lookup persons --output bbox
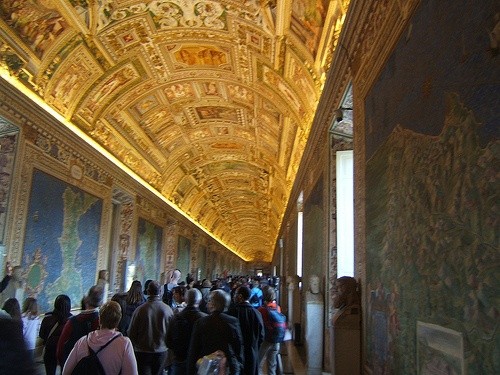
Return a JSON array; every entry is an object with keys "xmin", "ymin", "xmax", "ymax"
[
  {"xmin": 97, "ymin": 270, "xmax": 109, "ymax": 304},
  {"xmin": 9, "ymin": 266, "xmax": 25, "ymax": 312},
  {"xmin": 0, "ymin": 262, "xmax": 286, "ymax": 375},
  {"xmin": 330, "ymin": 276, "xmax": 362, "ymax": 375},
  {"xmin": 286, "ymin": 275, "xmax": 301, "ymax": 328}
]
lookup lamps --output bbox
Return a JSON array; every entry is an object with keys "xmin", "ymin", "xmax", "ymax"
[{"xmin": 336, "ymin": 105, "xmax": 353, "ymax": 121}]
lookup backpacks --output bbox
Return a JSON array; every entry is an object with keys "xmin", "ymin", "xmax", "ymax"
[
  {"xmin": 250, "ymin": 289, "xmax": 260, "ymax": 303},
  {"xmin": 63, "ymin": 315, "xmax": 97, "ymax": 361},
  {"xmin": 70, "ymin": 333, "xmax": 122, "ymax": 374},
  {"xmin": 263, "ymin": 303, "xmax": 285, "ymax": 344},
  {"xmin": 162, "ymin": 283, "xmax": 175, "ymax": 307}
]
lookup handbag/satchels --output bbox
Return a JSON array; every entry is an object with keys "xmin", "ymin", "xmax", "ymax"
[{"xmin": 40, "ymin": 345, "xmax": 46, "ymax": 363}]
